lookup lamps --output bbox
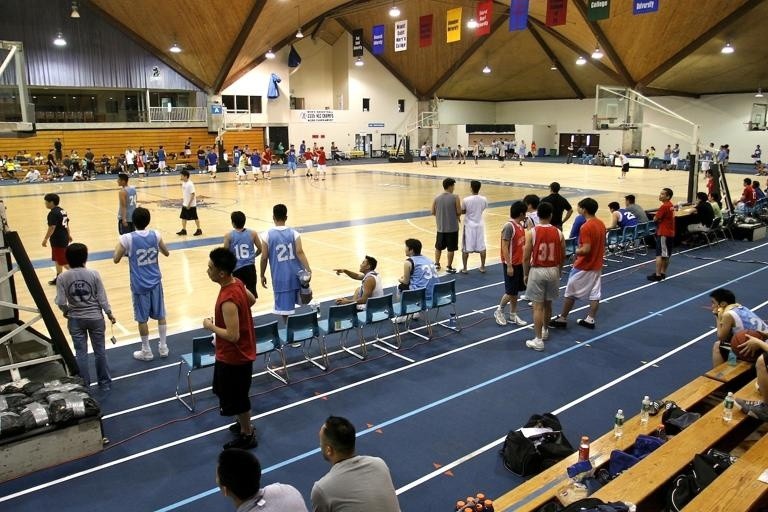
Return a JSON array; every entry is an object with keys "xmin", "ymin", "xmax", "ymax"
[{"xmin": 556, "ymin": 194, "xmax": 767, "ymax": 274}]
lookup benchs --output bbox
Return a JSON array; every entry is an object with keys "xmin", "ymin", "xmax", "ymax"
[
  {"xmin": 0, "ymin": 125, "xmax": 267, "ymax": 180},
  {"xmin": 644, "ymin": 158, "xmax": 764, "ymax": 176}
]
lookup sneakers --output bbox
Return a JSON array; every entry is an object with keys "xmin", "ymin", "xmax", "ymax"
[
  {"xmin": 133, "ymin": 349, "xmax": 153, "ymax": 361},
  {"xmin": 460, "ymin": 267, "xmax": 487, "ymax": 274},
  {"xmin": 494, "ymin": 294, "xmax": 595, "ymax": 352},
  {"xmin": 159, "ymin": 344, "xmax": 169, "ymax": 357},
  {"xmin": 176, "ymin": 229, "xmax": 187, "ymax": 236},
  {"xmin": 159, "ymin": 172, "xmax": 169, "ymax": 175},
  {"xmin": 647, "ymin": 273, "xmax": 665, "ymax": 282},
  {"xmin": 434, "ymin": 263, "xmax": 456, "ymax": 274},
  {"xmin": 48, "ymin": 273, "xmax": 59, "ymax": 285},
  {"xmin": 222, "ymin": 419, "xmax": 258, "ymax": 451},
  {"xmin": 199, "ymin": 169, "xmax": 327, "ymax": 185},
  {"xmin": 193, "ymin": 229, "xmax": 202, "ymax": 236},
  {"xmin": 390, "ymin": 312, "xmax": 420, "ymax": 324}
]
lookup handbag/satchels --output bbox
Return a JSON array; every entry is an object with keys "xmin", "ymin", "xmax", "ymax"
[{"xmin": 502, "ymin": 413, "xmax": 576, "ymax": 477}]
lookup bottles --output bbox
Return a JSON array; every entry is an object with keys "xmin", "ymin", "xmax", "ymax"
[
  {"xmin": 722, "ymin": 392, "xmax": 734, "ymax": 421},
  {"xmin": 578, "ymin": 436, "xmax": 590, "ymax": 462},
  {"xmin": 640, "ymin": 395, "xmax": 650, "ymax": 425},
  {"xmin": 728, "ymin": 349, "xmax": 736, "ymax": 365},
  {"xmin": 613, "ymin": 408, "xmax": 625, "ymax": 438}
]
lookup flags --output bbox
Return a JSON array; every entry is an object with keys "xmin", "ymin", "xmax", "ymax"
[{"xmin": 351, "ymin": 0, "xmax": 659, "ymax": 58}]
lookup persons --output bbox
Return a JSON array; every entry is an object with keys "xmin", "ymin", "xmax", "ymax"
[
  {"xmin": 569, "ymin": 201, "xmax": 586, "ymax": 246},
  {"xmin": 175, "ymin": 170, "xmax": 203, "ymax": 236},
  {"xmin": 41, "ymin": 192, "xmax": 74, "ymax": 286},
  {"xmin": 112, "ymin": 206, "xmax": 170, "ymax": 361},
  {"xmin": 734, "ymin": 329, "xmax": 768, "ymax": 423},
  {"xmin": 215, "ymin": 447, "xmax": 309, "ymax": 512},
  {"xmin": 751, "ymin": 145, "xmax": 768, "ymax": 176},
  {"xmin": 550, "ymin": 197, "xmax": 607, "ymax": 329},
  {"xmin": 734, "ymin": 178, "xmax": 768, "ymax": 214},
  {"xmin": 680, "ymin": 170, "xmax": 726, "ymax": 246},
  {"xmin": 461, "ymin": 180, "xmax": 488, "ymax": 274},
  {"xmin": 260, "ymin": 203, "xmax": 313, "ymax": 348},
  {"xmin": 708, "ymin": 288, "xmax": 768, "ymax": 370},
  {"xmin": 390, "ymin": 238, "xmax": 440, "ymax": 325},
  {"xmin": 309, "ymin": 414, "xmax": 402, "ymax": 511},
  {"xmin": 0, "ymin": 134, "xmax": 343, "ymax": 184},
  {"xmin": 222, "ymin": 210, "xmax": 264, "ymax": 299},
  {"xmin": 116, "ymin": 173, "xmax": 138, "ymax": 258},
  {"xmin": 54, "ymin": 242, "xmax": 117, "ymax": 391},
  {"xmin": 522, "ymin": 202, "xmax": 567, "ymax": 352},
  {"xmin": 431, "ymin": 177, "xmax": 462, "ymax": 274},
  {"xmin": 563, "ymin": 136, "xmax": 731, "ymax": 179},
  {"xmin": 645, "ymin": 188, "xmax": 676, "ymax": 282},
  {"xmin": 519, "ymin": 194, "xmax": 541, "ymax": 307},
  {"xmin": 418, "ymin": 135, "xmax": 536, "ymax": 169},
  {"xmin": 332, "ymin": 255, "xmax": 385, "ymax": 314},
  {"xmin": 493, "ymin": 200, "xmax": 530, "ymax": 327},
  {"xmin": 541, "ymin": 182, "xmax": 574, "ymax": 233},
  {"xmin": 624, "ymin": 194, "xmax": 650, "ymax": 232},
  {"xmin": 202, "ymin": 246, "xmax": 259, "ymax": 449},
  {"xmin": 604, "ymin": 201, "xmax": 638, "ymax": 237}
]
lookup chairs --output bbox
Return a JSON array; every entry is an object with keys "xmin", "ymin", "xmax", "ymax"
[
  {"xmin": 35, "ymin": 110, "xmax": 106, "ymax": 123},
  {"xmin": 570, "ymin": 149, "xmax": 618, "ymax": 167},
  {"xmin": 172, "ymin": 281, "xmax": 463, "ymax": 414}
]
